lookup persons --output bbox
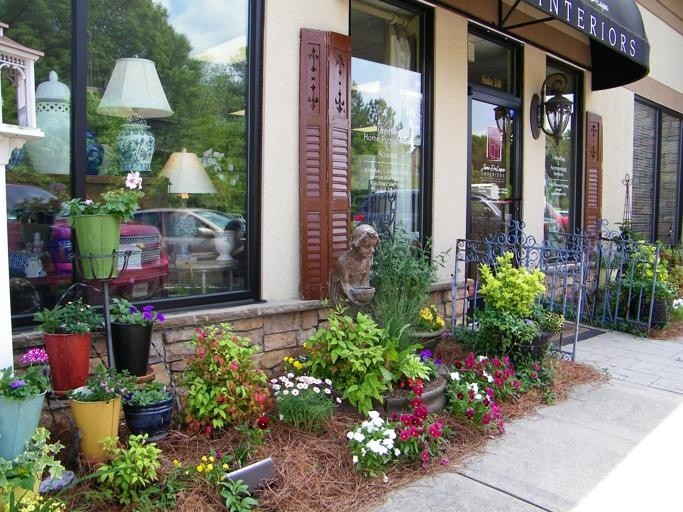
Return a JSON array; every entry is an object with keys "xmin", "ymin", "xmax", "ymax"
[
  {"xmin": 221, "ymin": 220, "xmax": 247, "ymax": 292},
  {"xmin": 329, "ymin": 223, "xmax": 381, "ymax": 320}
]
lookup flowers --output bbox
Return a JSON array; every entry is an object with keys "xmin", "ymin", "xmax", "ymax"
[
  {"xmin": 1, "ymin": 296, "xmax": 170, "ymax": 405},
  {"xmin": 10, "ymin": 182, "xmax": 68, "ymax": 243},
  {"xmin": 393, "ymin": 304, "xmax": 445, "ymax": 393},
  {"xmin": 61, "ymin": 171, "xmax": 146, "ymax": 222}
]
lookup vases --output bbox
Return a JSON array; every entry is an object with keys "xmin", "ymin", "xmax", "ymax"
[
  {"xmin": 2, "ymin": 388, "xmax": 47, "ymax": 460},
  {"xmin": 124, "ymin": 388, "xmax": 176, "ymax": 437},
  {"xmin": 69, "ymin": 398, "xmax": 121, "ymax": 462},
  {"xmin": 72, "ymin": 213, "xmax": 122, "ymax": 279},
  {"xmin": 381, "ymin": 358, "xmax": 449, "ymax": 419},
  {"xmin": 23, "ymin": 231, "xmax": 46, "ymax": 280}
]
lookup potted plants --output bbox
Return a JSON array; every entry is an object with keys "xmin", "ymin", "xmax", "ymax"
[
  {"xmin": 623, "ymin": 239, "xmax": 677, "ymax": 324},
  {"xmin": 476, "ymin": 251, "xmax": 566, "ymax": 366}
]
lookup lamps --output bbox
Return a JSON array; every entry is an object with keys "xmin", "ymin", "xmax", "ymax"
[
  {"xmin": 97, "ymin": 54, "xmax": 174, "ymax": 173},
  {"xmin": 530, "ymin": 72, "xmax": 574, "ymax": 147},
  {"xmin": 157, "ymin": 146, "xmax": 217, "ymax": 262}
]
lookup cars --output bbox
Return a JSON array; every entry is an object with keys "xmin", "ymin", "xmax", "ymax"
[
  {"xmin": 544, "ymin": 200, "xmax": 571, "ymax": 240},
  {"xmin": 363, "ymin": 188, "xmax": 421, "ymax": 243},
  {"xmin": 6, "ymin": 184, "xmax": 246, "ymax": 302},
  {"xmin": 466, "ymin": 183, "xmax": 509, "ymax": 242}
]
